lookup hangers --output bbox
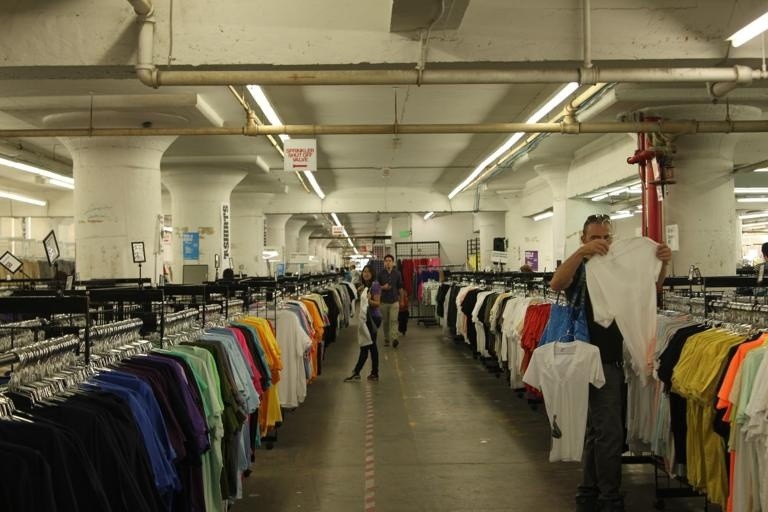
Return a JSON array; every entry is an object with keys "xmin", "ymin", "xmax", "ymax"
[
  {"xmin": 658, "ymin": 287, "xmax": 768, "ymax": 344},
  {"xmin": 598, "ymin": 217, "xmax": 625, "ymax": 250},
  {"xmin": 446, "ymin": 268, "xmax": 568, "ymax": 305},
  {"xmin": 158, "ymin": 309, "xmax": 204, "ymax": 345},
  {"xmin": 223, "ymin": 299, "xmax": 244, "ymax": 319},
  {"xmin": 0, "ymin": 332, "xmax": 100, "ymax": 424},
  {"xmin": 206, "ymin": 304, "xmax": 226, "ymax": 327},
  {"xmin": 551, "ymin": 317, "xmax": 579, "ymax": 358},
  {"xmin": 81, "ymin": 318, "xmax": 153, "ymax": 369}
]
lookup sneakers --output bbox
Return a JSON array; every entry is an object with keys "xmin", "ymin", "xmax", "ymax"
[
  {"xmin": 343, "ymin": 373, "xmax": 362, "ymax": 384},
  {"xmin": 391, "ymin": 338, "xmax": 400, "ymax": 349},
  {"xmin": 366, "ymin": 374, "xmax": 380, "ymax": 382}
]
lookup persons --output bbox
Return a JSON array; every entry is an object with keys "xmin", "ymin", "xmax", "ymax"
[
  {"xmin": 397, "ymin": 289, "xmax": 410, "ymax": 334},
  {"xmin": 520, "ymin": 265, "xmax": 533, "ymax": 272},
  {"xmin": 373, "ymin": 255, "xmax": 403, "ymax": 350},
  {"xmin": 752, "ymin": 242, "xmax": 768, "ymax": 274},
  {"xmin": 328, "ymin": 263, "xmax": 361, "ymax": 286},
  {"xmin": 343, "ymin": 263, "xmax": 381, "ymax": 382},
  {"xmin": 550, "ymin": 214, "xmax": 671, "ymax": 511}
]
